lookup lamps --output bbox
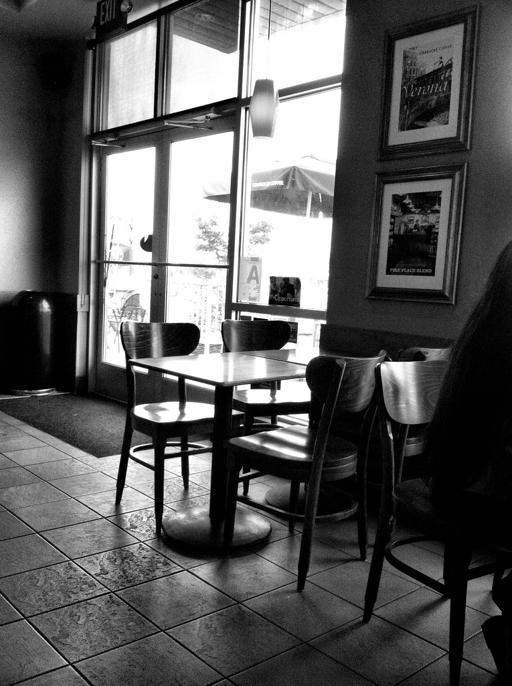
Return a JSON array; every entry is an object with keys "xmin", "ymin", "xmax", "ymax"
[{"xmin": 248, "ymin": 0, "xmax": 281, "ymax": 140}]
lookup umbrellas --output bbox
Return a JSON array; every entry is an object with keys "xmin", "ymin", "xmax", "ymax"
[{"xmin": 197, "ymin": 153, "xmax": 335, "ymax": 222}]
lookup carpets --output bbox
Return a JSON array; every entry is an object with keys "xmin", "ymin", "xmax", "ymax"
[{"xmin": 0, "ymin": 391, "xmax": 210, "ymax": 459}]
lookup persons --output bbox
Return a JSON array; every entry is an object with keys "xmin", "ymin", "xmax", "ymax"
[
  {"xmin": 270, "ymin": 276, "xmax": 300, "ymax": 298},
  {"xmin": 413, "ymin": 219, "xmax": 420, "ymax": 230},
  {"xmin": 419, "ymin": 236, "xmax": 512, "ymax": 686}
]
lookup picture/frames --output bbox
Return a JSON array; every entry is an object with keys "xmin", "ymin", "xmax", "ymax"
[{"xmin": 363, "ymin": 4, "xmax": 482, "ymax": 306}]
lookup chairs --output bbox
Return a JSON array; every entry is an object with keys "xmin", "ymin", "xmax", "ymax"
[{"xmin": 106, "ymin": 294, "xmax": 148, "ymax": 352}]
